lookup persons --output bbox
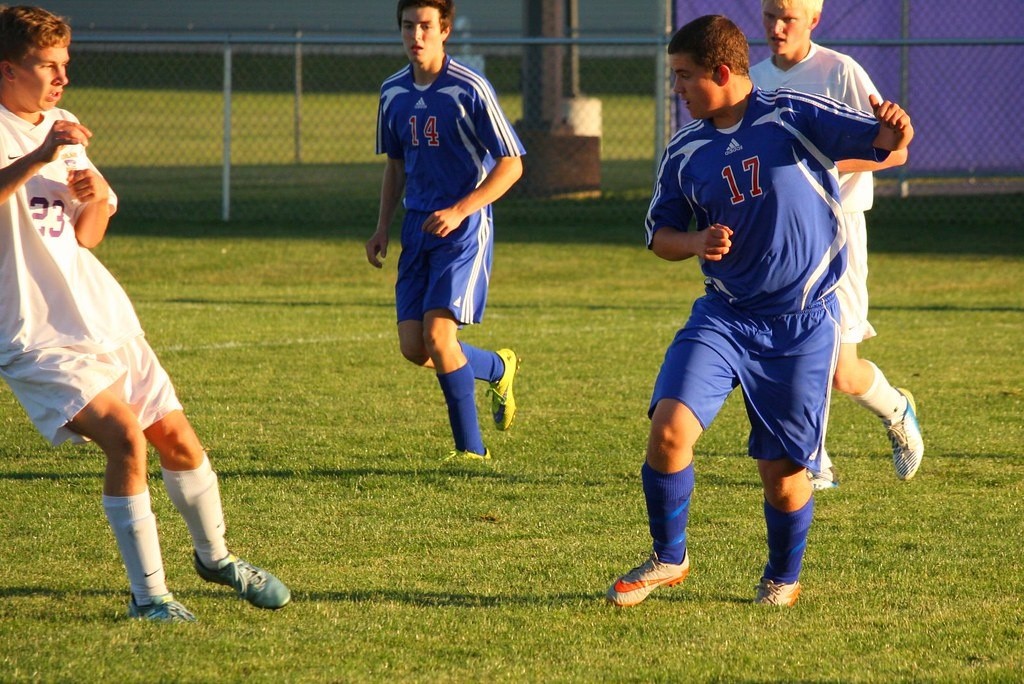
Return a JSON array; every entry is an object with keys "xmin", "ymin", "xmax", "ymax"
[
  {"xmin": 747, "ymin": 0, "xmax": 925, "ymax": 482},
  {"xmin": 364, "ymin": 0, "xmax": 527, "ymax": 469},
  {"xmin": 607, "ymin": 13, "xmax": 915, "ymax": 609},
  {"xmin": 1, "ymin": 5, "xmax": 292, "ymax": 623}
]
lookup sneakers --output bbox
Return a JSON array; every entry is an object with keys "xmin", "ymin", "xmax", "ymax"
[
  {"xmin": 811, "ymin": 448, "xmax": 839, "ymax": 491},
  {"xmin": 883, "ymin": 387, "xmax": 925, "ymax": 480},
  {"xmin": 439, "ymin": 448, "xmax": 492, "ymax": 461},
  {"xmin": 607, "ymin": 549, "xmax": 690, "ymax": 607},
  {"xmin": 193, "ymin": 548, "xmax": 290, "ymax": 609},
  {"xmin": 754, "ymin": 575, "xmax": 801, "ymax": 605},
  {"xmin": 128, "ymin": 593, "xmax": 196, "ymax": 623},
  {"xmin": 485, "ymin": 348, "xmax": 520, "ymax": 432}
]
